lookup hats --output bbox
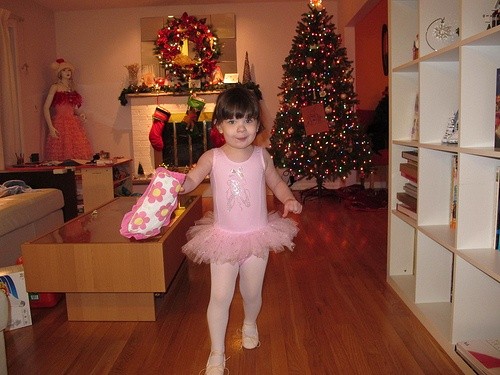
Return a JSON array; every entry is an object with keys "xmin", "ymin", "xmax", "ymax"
[{"xmin": 57, "ymin": 62, "xmax": 74, "ymax": 79}]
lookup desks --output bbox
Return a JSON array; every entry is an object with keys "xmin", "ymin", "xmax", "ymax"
[
  {"xmin": 360, "ymin": 164, "xmax": 388, "ymax": 199},
  {"xmin": 0, "ymin": 163, "xmax": 112, "ymax": 217},
  {"xmin": 22, "ymin": 196, "xmax": 202, "ymax": 321}
]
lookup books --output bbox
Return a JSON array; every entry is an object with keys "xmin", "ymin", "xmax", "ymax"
[
  {"xmin": 450, "ymin": 154, "xmax": 458, "ymax": 229},
  {"xmin": 494, "ymin": 68, "xmax": 500, "ymax": 148},
  {"xmin": 454, "ymin": 337, "xmax": 500, "ymax": 375},
  {"xmin": 493, "ymin": 166, "xmax": 500, "ymax": 251},
  {"xmin": 396, "ymin": 147, "xmax": 418, "ymax": 221}
]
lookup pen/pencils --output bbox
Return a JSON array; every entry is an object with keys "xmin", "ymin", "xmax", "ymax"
[{"xmin": 15, "ymin": 152, "xmax": 25, "ymax": 157}]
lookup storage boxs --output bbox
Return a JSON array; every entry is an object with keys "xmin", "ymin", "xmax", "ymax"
[{"xmin": 0, "ymin": 258, "xmax": 59, "ymax": 331}]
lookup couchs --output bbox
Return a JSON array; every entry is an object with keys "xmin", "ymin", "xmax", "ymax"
[{"xmin": 0, "ymin": 188, "xmax": 64, "ymax": 268}]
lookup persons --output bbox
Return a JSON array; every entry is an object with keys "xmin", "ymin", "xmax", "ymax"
[
  {"xmin": 43, "ymin": 58, "xmax": 95, "ymax": 160},
  {"xmin": 177, "ymin": 81, "xmax": 303, "ymax": 375}
]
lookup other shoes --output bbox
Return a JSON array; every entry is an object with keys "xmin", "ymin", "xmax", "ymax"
[
  {"xmin": 205, "ymin": 351, "xmax": 226, "ymax": 375},
  {"xmin": 242, "ymin": 323, "xmax": 258, "ymax": 349}
]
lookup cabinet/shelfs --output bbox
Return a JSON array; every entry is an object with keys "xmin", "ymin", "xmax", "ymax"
[
  {"xmin": 130, "ymin": 88, "xmax": 221, "ymax": 196},
  {"xmin": 111, "ymin": 156, "xmax": 133, "ymax": 195},
  {"xmin": 387, "ymin": 0, "xmax": 500, "ymax": 375}
]
